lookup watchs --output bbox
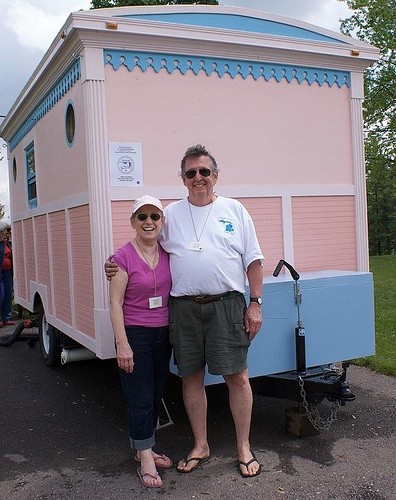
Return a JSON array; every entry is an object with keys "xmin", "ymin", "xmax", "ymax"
[{"xmin": 249, "ymin": 297, "xmax": 262, "ymax": 305}]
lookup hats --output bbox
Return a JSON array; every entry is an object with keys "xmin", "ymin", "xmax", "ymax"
[{"xmin": 131, "ymin": 195, "xmax": 162, "ymax": 213}]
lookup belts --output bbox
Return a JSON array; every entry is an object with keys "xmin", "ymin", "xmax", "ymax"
[{"xmin": 179, "ymin": 291, "xmax": 238, "ymax": 303}]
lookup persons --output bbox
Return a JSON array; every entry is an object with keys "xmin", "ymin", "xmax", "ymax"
[
  {"xmin": 105, "ymin": 145, "xmax": 265, "ymax": 478},
  {"xmin": 109, "ymin": 195, "xmax": 172, "ymax": 487},
  {"xmin": 0, "ymin": 218, "xmax": 16, "ymax": 327}
]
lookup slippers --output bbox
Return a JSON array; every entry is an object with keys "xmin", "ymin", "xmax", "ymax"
[
  {"xmin": 136, "ymin": 466, "xmax": 163, "ymax": 488},
  {"xmin": 237, "ymin": 449, "xmax": 260, "ymax": 477},
  {"xmin": 175, "ymin": 449, "xmax": 212, "ymax": 473},
  {"xmin": 133, "ymin": 452, "xmax": 172, "ymax": 467}
]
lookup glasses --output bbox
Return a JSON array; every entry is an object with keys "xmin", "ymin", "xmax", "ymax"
[
  {"xmin": 137, "ymin": 214, "xmax": 160, "ymax": 220},
  {"xmin": 184, "ymin": 168, "xmax": 210, "ymax": 179}
]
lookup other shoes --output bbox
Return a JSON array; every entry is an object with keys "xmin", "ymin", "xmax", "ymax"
[{"xmin": 0, "ymin": 320, "xmax": 15, "ymax": 326}]
